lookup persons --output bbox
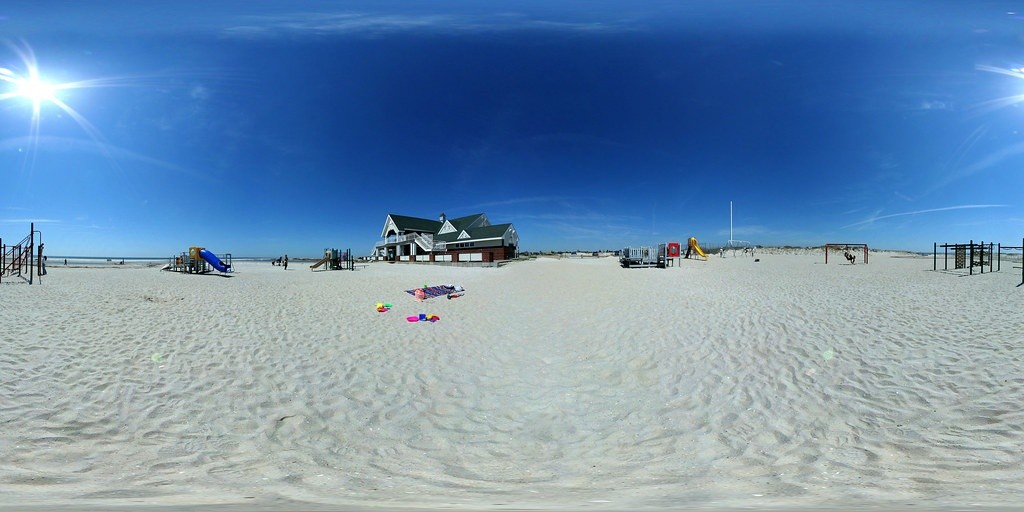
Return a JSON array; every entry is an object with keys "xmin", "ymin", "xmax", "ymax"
[
  {"xmin": 845, "ymin": 248, "xmax": 856, "ymax": 263},
  {"xmin": 42, "ymin": 255, "xmax": 47, "ymax": 275},
  {"xmin": 64, "ymin": 259, "xmax": 68, "ymax": 265},
  {"xmin": 277, "ymin": 257, "xmax": 282, "ymax": 266},
  {"xmin": 284, "ymin": 255, "xmax": 288, "ymax": 270},
  {"xmin": 719, "ymin": 247, "xmax": 757, "ymax": 258}
]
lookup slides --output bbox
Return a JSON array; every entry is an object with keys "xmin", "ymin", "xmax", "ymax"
[
  {"xmin": 694, "ymin": 244, "xmax": 709, "ymax": 257},
  {"xmin": 310, "ymin": 258, "xmax": 329, "ymax": 268},
  {"xmin": 199, "ymin": 249, "xmax": 231, "ymax": 272}
]
